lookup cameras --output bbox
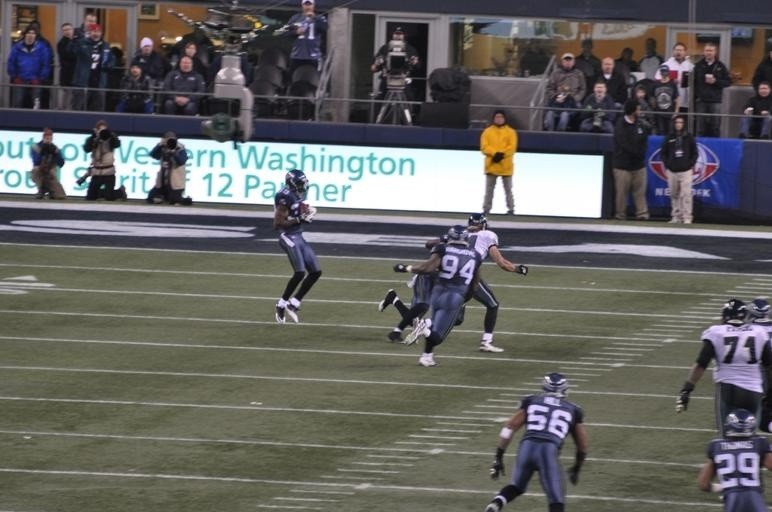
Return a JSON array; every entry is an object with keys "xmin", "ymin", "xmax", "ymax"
[
  {"xmin": 40, "ymin": 143, "xmax": 54, "ymax": 154},
  {"xmin": 96, "ymin": 128, "xmax": 112, "ymax": 143},
  {"xmin": 76, "ymin": 172, "xmax": 91, "ymax": 186},
  {"xmin": 167, "ymin": 137, "xmax": 178, "ymax": 150}
]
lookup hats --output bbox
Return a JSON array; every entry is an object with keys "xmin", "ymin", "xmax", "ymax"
[
  {"xmin": 140, "ymin": 36, "xmax": 154, "ymax": 49},
  {"xmin": 658, "ymin": 65, "xmax": 670, "ymax": 73},
  {"xmin": 41, "ymin": 126, "xmax": 53, "ymax": 136},
  {"xmin": 491, "ymin": 109, "xmax": 507, "ymax": 119},
  {"xmin": 95, "ymin": 119, "xmax": 108, "ymax": 128},
  {"xmin": 391, "ymin": 25, "xmax": 404, "ymax": 35},
  {"xmin": 163, "ymin": 131, "xmax": 177, "ymax": 140},
  {"xmin": 559, "ymin": 53, "xmax": 575, "ymax": 60},
  {"xmin": 88, "ymin": 23, "xmax": 102, "ymax": 33},
  {"xmin": 300, "ymin": 0, "xmax": 315, "ymax": 5}
]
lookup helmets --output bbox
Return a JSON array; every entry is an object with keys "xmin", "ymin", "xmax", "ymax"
[
  {"xmin": 541, "ymin": 370, "xmax": 569, "ymax": 392},
  {"xmin": 448, "ymin": 225, "xmax": 466, "ymax": 244},
  {"xmin": 722, "ymin": 299, "xmax": 748, "ymax": 323},
  {"xmin": 465, "ymin": 212, "xmax": 488, "ymax": 225},
  {"xmin": 748, "ymin": 298, "xmax": 771, "ymax": 319},
  {"xmin": 722, "ymin": 407, "xmax": 756, "ymax": 437},
  {"xmin": 285, "ymin": 170, "xmax": 310, "ymax": 199}
]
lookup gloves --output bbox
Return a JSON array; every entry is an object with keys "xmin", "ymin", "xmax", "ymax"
[
  {"xmin": 493, "ymin": 151, "xmax": 504, "ymax": 162},
  {"xmin": 490, "ymin": 460, "xmax": 507, "ymax": 478},
  {"xmin": 517, "ymin": 262, "xmax": 529, "ymax": 277},
  {"xmin": 302, "ymin": 211, "xmax": 316, "ymax": 223},
  {"xmin": 567, "ymin": 465, "xmax": 578, "ymax": 487},
  {"xmin": 392, "ymin": 262, "xmax": 408, "ymax": 273},
  {"xmin": 675, "ymin": 392, "xmax": 689, "ymax": 414},
  {"xmin": 301, "ymin": 202, "xmax": 317, "ymax": 211}
]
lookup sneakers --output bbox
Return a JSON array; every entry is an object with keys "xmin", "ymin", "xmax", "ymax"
[
  {"xmin": 480, "ymin": 211, "xmax": 490, "ymax": 217},
  {"xmin": 286, "ymin": 299, "xmax": 300, "ymax": 325},
  {"xmin": 505, "ymin": 210, "xmax": 515, "ymax": 215},
  {"xmin": 484, "ymin": 496, "xmax": 502, "ymax": 511},
  {"xmin": 120, "ymin": 185, "xmax": 128, "ymax": 201},
  {"xmin": 388, "ymin": 330, "xmax": 405, "ymax": 346},
  {"xmin": 184, "ymin": 196, "xmax": 193, "ymax": 206},
  {"xmin": 377, "ymin": 286, "xmax": 397, "ymax": 313},
  {"xmin": 403, "ymin": 318, "xmax": 429, "ymax": 346},
  {"xmin": 667, "ymin": 217, "xmax": 681, "ymax": 224},
  {"xmin": 274, "ymin": 303, "xmax": 286, "ymax": 324},
  {"xmin": 682, "ymin": 218, "xmax": 691, "ymax": 225},
  {"xmin": 611, "ymin": 215, "xmax": 627, "ymax": 220},
  {"xmin": 480, "ymin": 338, "xmax": 504, "ymax": 354},
  {"xmin": 633, "ymin": 214, "xmax": 650, "ymax": 221},
  {"xmin": 419, "ymin": 355, "xmax": 442, "ymax": 366},
  {"xmin": 48, "ymin": 191, "xmax": 52, "ymax": 200},
  {"xmin": 35, "ymin": 193, "xmax": 46, "ymax": 199}
]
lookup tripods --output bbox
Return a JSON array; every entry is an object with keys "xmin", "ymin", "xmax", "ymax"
[{"xmin": 376, "ymin": 90, "xmax": 413, "ymax": 125}]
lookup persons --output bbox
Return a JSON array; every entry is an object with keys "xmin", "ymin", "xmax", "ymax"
[
  {"xmin": 379, "ymin": 235, "xmax": 456, "ymax": 344},
  {"xmin": 29, "ymin": 127, "xmax": 68, "ymax": 199},
  {"xmin": 72, "ymin": 25, "xmax": 115, "ymax": 111},
  {"xmin": 7, "ymin": 26, "xmax": 50, "ymax": 108},
  {"xmin": 676, "ymin": 300, "xmax": 771, "ymax": 439},
  {"xmin": 589, "ymin": 57, "xmax": 628, "ymax": 107},
  {"xmin": 633, "ymin": 83, "xmax": 655, "ymax": 133},
  {"xmin": 660, "ymin": 116, "xmax": 698, "ymax": 224},
  {"xmin": 274, "ymin": 170, "xmax": 322, "ymax": 326},
  {"xmin": 163, "ymin": 56, "xmax": 206, "ymax": 115},
  {"xmin": 370, "ymin": 25, "xmax": 419, "ymax": 115},
  {"xmin": 746, "ymin": 294, "xmax": 771, "ymax": 431},
  {"xmin": 638, "ymin": 38, "xmax": 665, "ymax": 75},
  {"xmin": 9, "ymin": 20, "xmax": 54, "ymax": 108},
  {"xmin": 57, "ymin": 22, "xmax": 76, "ymax": 87},
  {"xmin": 425, "ymin": 214, "xmax": 528, "ymax": 353},
  {"xmin": 132, "ymin": 37, "xmax": 166, "ymax": 82},
  {"xmin": 65, "ymin": 13, "xmax": 99, "ymax": 69},
  {"xmin": 147, "ymin": 132, "xmax": 194, "ymax": 205},
  {"xmin": 695, "ymin": 41, "xmax": 732, "ymax": 136},
  {"xmin": 393, "ymin": 225, "xmax": 481, "ymax": 367},
  {"xmin": 614, "ymin": 48, "xmax": 640, "ymax": 70},
  {"xmin": 168, "ymin": 41, "xmax": 206, "ymax": 79},
  {"xmin": 486, "ymin": 374, "xmax": 588, "ymax": 512},
  {"xmin": 612, "ymin": 100, "xmax": 652, "ymax": 221},
  {"xmin": 542, "ymin": 52, "xmax": 587, "ymax": 131},
  {"xmin": 739, "ymin": 82, "xmax": 770, "ymax": 138},
  {"xmin": 481, "ymin": 110, "xmax": 519, "ymax": 215},
  {"xmin": 699, "ymin": 408, "xmax": 772, "ymax": 512},
  {"xmin": 751, "ymin": 49, "xmax": 771, "ymax": 89},
  {"xmin": 652, "ymin": 65, "xmax": 680, "ymax": 135},
  {"xmin": 84, "ymin": 119, "xmax": 127, "ymax": 199},
  {"xmin": 580, "ymin": 80, "xmax": 617, "ymax": 132},
  {"xmin": 573, "ymin": 39, "xmax": 602, "ymax": 79},
  {"xmin": 212, "ymin": 52, "xmax": 253, "ymax": 87},
  {"xmin": 661, "ymin": 41, "xmax": 696, "ymax": 131},
  {"xmin": 115, "ymin": 57, "xmax": 155, "ymax": 113},
  {"xmin": 272, "ymin": 0, "xmax": 328, "ymax": 109}
]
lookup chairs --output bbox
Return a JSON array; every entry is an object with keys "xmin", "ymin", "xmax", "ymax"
[{"xmin": 198, "ymin": 33, "xmax": 323, "ymax": 122}]
